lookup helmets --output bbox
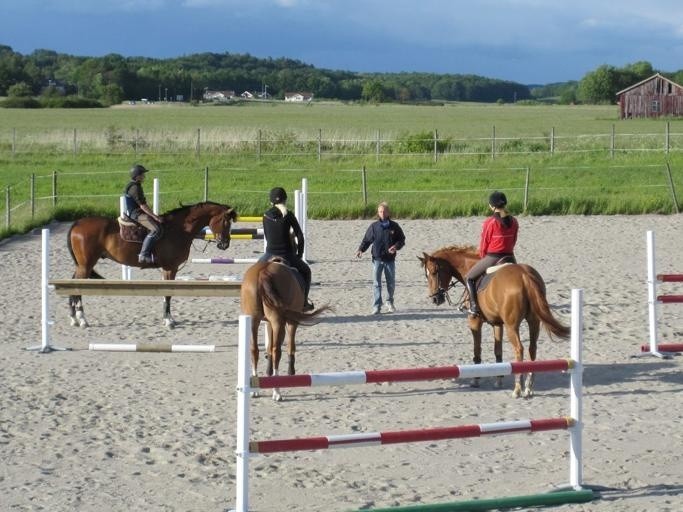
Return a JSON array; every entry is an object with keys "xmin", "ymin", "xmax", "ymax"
[
  {"xmin": 129, "ymin": 164, "xmax": 149, "ymax": 178},
  {"xmin": 269, "ymin": 186, "xmax": 287, "ymax": 204}
]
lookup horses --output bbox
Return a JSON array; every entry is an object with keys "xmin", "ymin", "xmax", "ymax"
[
  {"xmin": 66, "ymin": 199, "xmax": 238, "ymax": 330},
  {"xmin": 415, "ymin": 244, "xmax": 571, "ymax": 400},
  {"xmin": 240, "ymin": 230, "xmax": 337, "ymax": 402}
]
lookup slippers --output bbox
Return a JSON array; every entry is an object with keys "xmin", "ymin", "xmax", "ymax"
[{"xmin": 464, "ymin": 278, "xmax": 479, "ymax": 318}]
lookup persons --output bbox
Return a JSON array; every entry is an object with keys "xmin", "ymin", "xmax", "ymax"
[
  {"xmin": 353, "ymin": 203, "xmax": 405, "ymax": 316},
  {"xmin": 123, "ymin": 163, "xmax": 164, "ymax": 265},
  {"xmin": 465, "ymin": 192, "xmax": 519, "ymax": 317},
  {"xmin": 263, "ymin": 187, "xmax": 314, "ymax": 310}
]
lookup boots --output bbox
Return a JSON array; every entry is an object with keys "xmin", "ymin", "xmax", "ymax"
[
  {"xmin": 304, "ymin": 279, "xmax": 314, "ymax": 311},
  {"xmin": 137, "ymin": 233, "xmax": 153, "ymax": 264}
]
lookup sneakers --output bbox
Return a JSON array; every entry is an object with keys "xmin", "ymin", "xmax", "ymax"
[
  {"xmin": 372, "ymin": 307, "xmax": 381, "ymax": 316},
  {"xmin": 385, "ymin": 301, "xmax": 396, "ymax": 313}
]
